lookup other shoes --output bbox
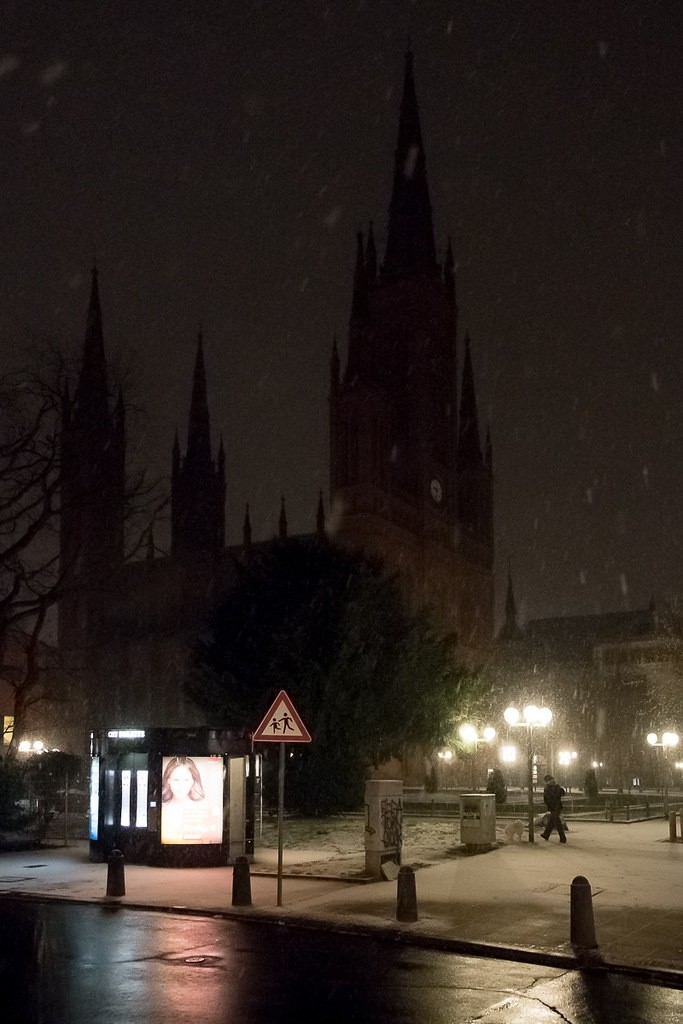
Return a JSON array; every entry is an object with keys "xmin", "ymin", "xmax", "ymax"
[
  {"xmin": 559, "ymin": 838, "xmax": 566, "ymax": 843},
  {"xmin": 540, "ymin": 834, "xmax": 549, "ymax": 840}
]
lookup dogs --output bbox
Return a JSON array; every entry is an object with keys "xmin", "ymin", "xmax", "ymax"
[{"xmin": 504, "ymin": 820, "xmax": 524, "ymax": 843}]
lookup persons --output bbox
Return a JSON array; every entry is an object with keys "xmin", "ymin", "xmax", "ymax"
[{"xmin": 539, "ymin": 775, "xmax": 568, "ymax": 845}]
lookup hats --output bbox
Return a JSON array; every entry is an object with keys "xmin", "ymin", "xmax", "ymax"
[{"xmin": 543, "ymin": 775, "xmax": 553, "ymax": 781}]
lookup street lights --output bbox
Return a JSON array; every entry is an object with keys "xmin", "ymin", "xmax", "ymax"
[
  {"xmin": 648, "ymin": 733, "xmax": 678, "ymax": 818},
  {"xmin": 462, "ymin": 722, "xmax": 496, "ymax": 789},
  {"xmin": 501, "ymin": 705, "xmax": 554, "ymax": 844}
]
